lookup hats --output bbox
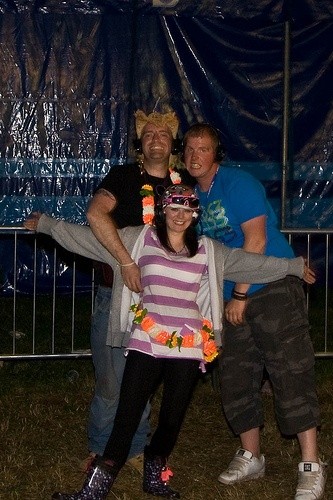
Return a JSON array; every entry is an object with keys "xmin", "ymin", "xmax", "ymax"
[{"xmin": 132, "ymin": 109, "xmax": 180, "ymax": 140}]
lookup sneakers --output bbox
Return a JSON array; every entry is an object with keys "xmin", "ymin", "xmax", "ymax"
[
  {"xmin": 294, "ymin": 456, "xmax": 327, "ymax": 500},
  {"xmin": 217, "ymin": 449, "xmax": 264, "ymax": 485}
]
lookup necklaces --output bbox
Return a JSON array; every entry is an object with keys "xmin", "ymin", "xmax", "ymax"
[
  {"xmin": 136, "ymin": 153, "xmax": 182, "ymax": 224},
  {"xmin": 167, "ymin": 233, "xmax": 186, "ymax": 254},
  {"xmin": 194, "ymin": 163, "xmax": 219, "ymax": 234}
]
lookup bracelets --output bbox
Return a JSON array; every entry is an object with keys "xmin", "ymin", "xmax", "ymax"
[
  {"xmin": 117, "ymin": 260, "xmax": 135, "ymax": 267},
  {"xmin": 231, "ymin": 288, "xmax": 247, "ymax": 301}
]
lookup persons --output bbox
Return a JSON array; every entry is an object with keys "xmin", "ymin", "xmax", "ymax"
[
  {"xmin": 186, "ymin": 123, "xmax": 328, "ymax": 500},
  {"xmin": 80, "ymin": 112, "xmax": 197, "ymax": 482},
  {"xmin": 23, "ymin": 183, "xmax": 315, "ymax": 500}
]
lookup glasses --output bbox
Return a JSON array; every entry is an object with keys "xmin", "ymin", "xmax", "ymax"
[{"xmin": 162, "ymin": 195, "xmax": 200, "ymax": 208}]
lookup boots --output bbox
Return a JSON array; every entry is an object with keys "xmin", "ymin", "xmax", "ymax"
[
  {"xmin": 143, "ymin": 454, "xmax": 180, "ymax": 498},
  {"xmin": 53, "ymin": 463, "xmax": 117, "ymax": 499}
]
